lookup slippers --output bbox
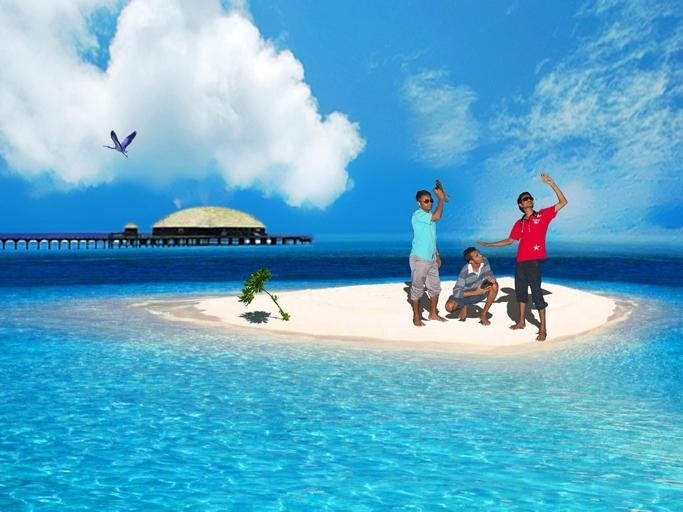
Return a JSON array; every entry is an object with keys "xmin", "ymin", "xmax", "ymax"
[{"xmin": 435, "ymin": 179, "xmax": 449, "ymax": 203}]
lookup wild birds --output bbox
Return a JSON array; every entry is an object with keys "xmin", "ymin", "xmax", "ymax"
[{"xmin": 103, "ymin": 130, "xmax": 136, "ymax": 159}]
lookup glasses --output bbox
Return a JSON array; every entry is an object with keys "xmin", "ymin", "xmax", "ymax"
[
  {"xmin": 419, "ymin": 199, "xmax": 433, "ymax": 204},
  {"xmin": 519, "ymin": 196, "xmax": 534, "ymax": 203}
]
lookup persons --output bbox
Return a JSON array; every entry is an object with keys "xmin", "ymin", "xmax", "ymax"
[
  {"xmin": 445, "ymin": 247, "xmax": 498, "ymax": 326},
  {"xmin": 477, "ymin": 173, "xmax": 568, "ymax": 341},
  {"xmin": 409, "ymin": 189, "xmax": 448, "ymax": 326}
]
